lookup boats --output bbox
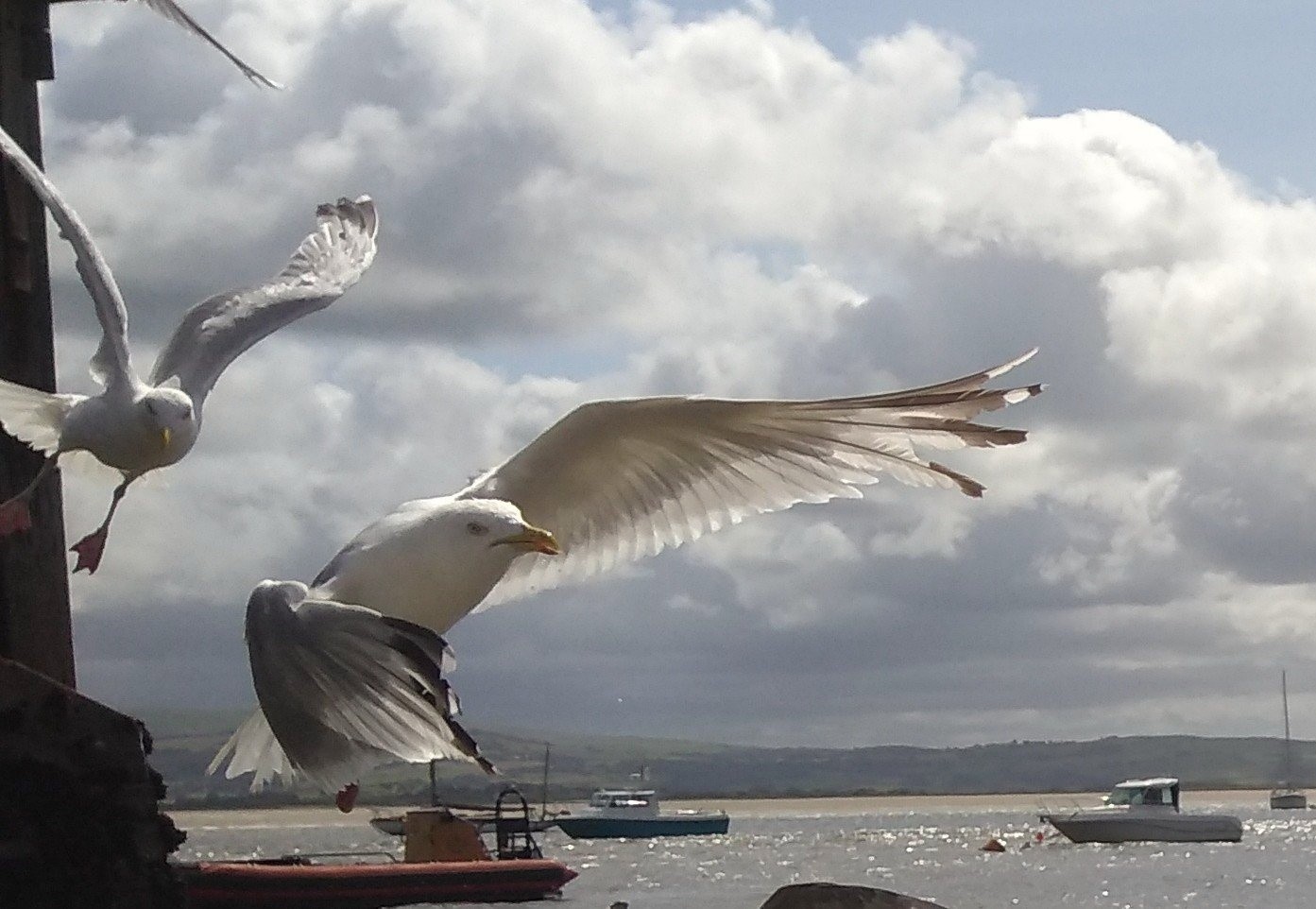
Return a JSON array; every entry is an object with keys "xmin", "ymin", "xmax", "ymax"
[
  {"xmin": 1037, "ymin": 777, "xmax": 1248, "ymax": 845},
  {"xmin": 551, "ymin": 766, "xmax": 731, "ymax": 840},
  {"xmin": 179, "ymin": 784, "xmax": 583, "ymax": 909}
]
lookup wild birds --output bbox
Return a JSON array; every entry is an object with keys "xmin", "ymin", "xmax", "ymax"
[
  {"xmin": 0, "ymin": 118, "xmax": 385, "ymax": 581},
  {"xmin": 198, "ymin": 340, "xmax": 1056, "ymax": 816},
  {"xmin": 1, "ymin": 0, "xmax": 287, "ymax": 94}
]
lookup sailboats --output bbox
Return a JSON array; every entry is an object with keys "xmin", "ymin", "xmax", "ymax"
[
  {"xmin": 368, "ymin": 756, "xmax": 557, "ymax": 841},
  {"xmin": 1268, "ymin": 670, "xmax": 1316, "ymax": 812}
]
